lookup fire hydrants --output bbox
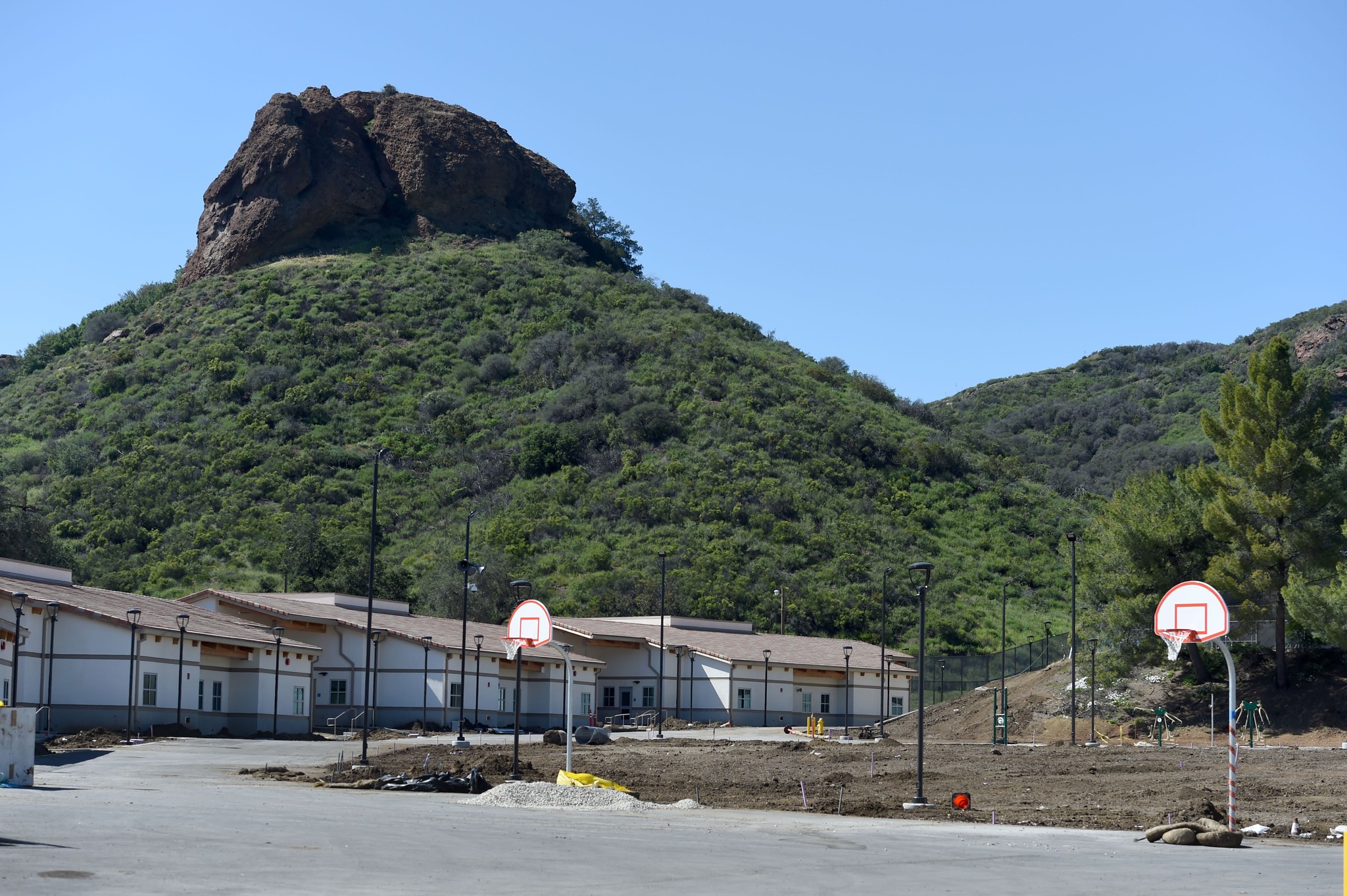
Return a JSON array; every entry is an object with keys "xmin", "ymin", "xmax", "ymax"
[{"xmin": 816, "ymin": 718, "xmax": 825, "ymax": 735}]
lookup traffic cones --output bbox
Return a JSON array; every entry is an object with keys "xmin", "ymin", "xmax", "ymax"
[
  {"xmin": 951, "ymin": 792, "xmax": 971, "ymax": 811},
  {"xmin": 720, "ymin": 720, "xmax": 732, "ymax": 728}
]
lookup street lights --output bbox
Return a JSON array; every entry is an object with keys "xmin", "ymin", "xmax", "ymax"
[
  {"xmin": 1044, "ymin": 621, "xmax": 1052, "ymax": 666},
  {"xmin": 762, "ymin": 649, "xmax": 772, "ymax": 728},
  {"xmin": 906, "ymin": 562, "xmax": 946, "ymax": 804},
  {"xmin": 1000, "ymin": 579, "xmax": 1012, "ymax": 740},
  {"xmin": 1065, "ymin": 532, "xmax": 1100, "ymax": 745},
  {"xmin": 420, "ymin": 635, "xmax": 433, "ymax": 737},
  {"xmin": 877, "ymin": 566, "xmax": 894, "ymax": 739},
  {"xmin": 176, "ymin": 613, "xmax": 191, "ymax": 738},
  {"xmin": 673, "ymin": 646, "xmax": 698, "ymax": 724},
  {"xmin": 273, "ymin": 627, "xmax": 285, "ymax": 740},
  {"xmin": 902, "ymin": 647, "xmax": 910, "ymax": 667},
  {"xmin": 44, "ymin": 602, "xmax": 60, "ymax": 731},
  {"xmin": 126, "ymin": 609, "xmax": 142, "ymax": 746},
  {"xmin": 457, "ymin": 508, "xmax": 489, "ymax": 741},
  {"xmin": 509, "ymin": 580, "xmax": 534, "ymax": 781},
  {"xmin": 558, "ymin": 645, "xmax": 571, "ymax": 731},
  {"xmin": 1026, "ymin": 635, "xmax": 1035, "ymax": 672},
  {"xmin": 656, "ymin": 550, "xmax": 667, "ymax": 738},
  {"xmin": 359, "ymin": 446, "xmax": 393, "ymax": 765},
  {"xmin": 842, "ymin": 645, "xmax": 853, "ymax": 737},
  {"xmin": 773, "ymin": 588, "xmax": 785, "ymax": 635},
  {"xmin": 8, "ymin": 592, "xmax": 29, "ymax": 708}
]
